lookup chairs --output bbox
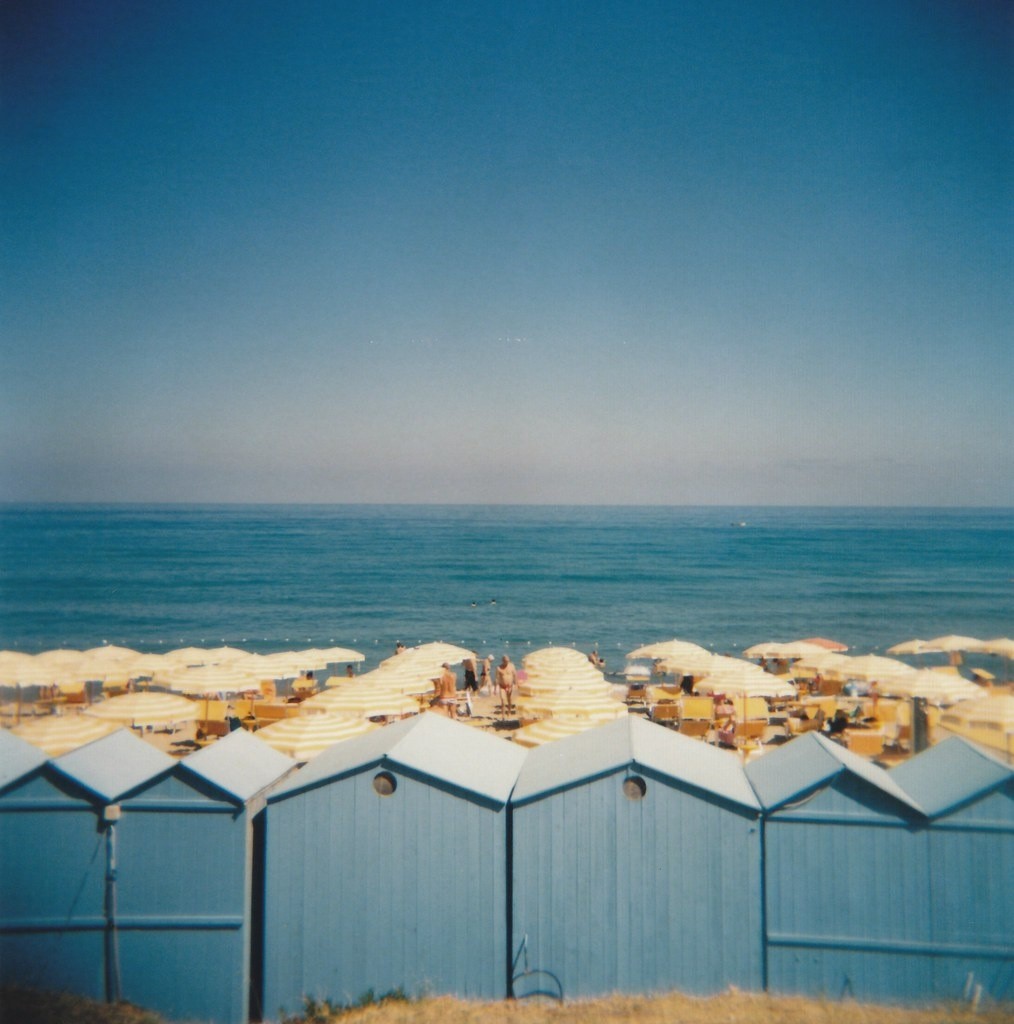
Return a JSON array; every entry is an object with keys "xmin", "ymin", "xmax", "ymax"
[{"xmin": 51, "ymin": 665, "xmax": 934, "ymax": 772}]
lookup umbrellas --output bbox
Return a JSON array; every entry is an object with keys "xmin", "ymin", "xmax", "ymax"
[{"xmin": 0, "ymin": 634, "xmax": 1014, "ymax": 763}]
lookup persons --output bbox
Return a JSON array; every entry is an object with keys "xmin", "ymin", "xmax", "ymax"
[
  {"xmin": 494, "ymin": 656, "xmax": 517, "ymax": 720},
  {"xmin": 440, "ymin": 662, "xmax": 458, "ymax": 719},
  {"xmin": 826, "ymin": 710, "xmax": 847, "ymax": 735},
  {"xmin": 489, "ymin": 600, "xmax": 496, "ymax": 606},
  {"xmin": 773, "ymin": 657, "xmax": 790, "ymax": 672},
  {"xmin": 589, "ymin": 650, "xmax": 597, "ymax": 664},
  {"xmin": 598, "ymin": 658, "xmax": 605, "ymax": 669},
  {"xmin": 462, "ymin": 659, "xmax": 478, "ymax": 717},
  {"xmin": 347, "ymin": 665, "xmax": 353, "ymax": 677},
  {"xmin": 760, "ymin": 656, "xmax": 767, "ymax": 672},
  {"xmin": 396, "ymin": 643, "xmax": 404, "ymax": 654},
  {"xmin": 471, "ymin": 602, "xmax": 478, "ymax": 607},
  {"xmin": 479, "ymin": 654, "xmax": 494, "ymax": 696},
  {"xmin": 225, "ymin": 705, "xmax": 241, "ymax": 732},
  {"xmin": 292, "ymin": 671, "xmax": 316, "ymax": 687}
]
